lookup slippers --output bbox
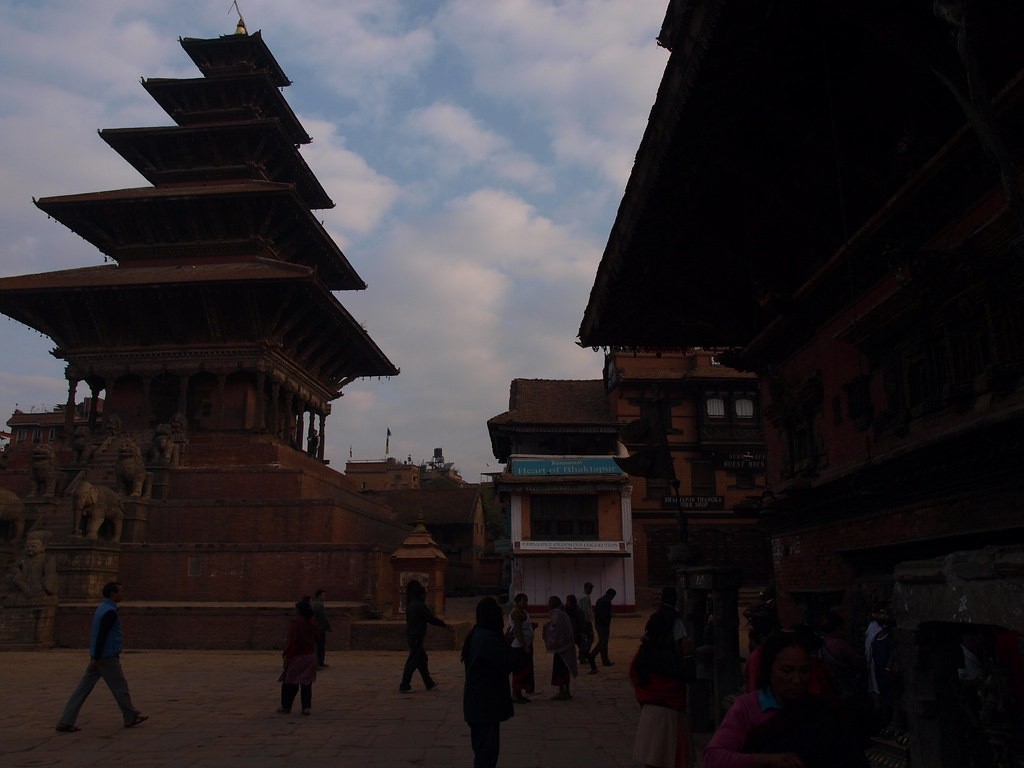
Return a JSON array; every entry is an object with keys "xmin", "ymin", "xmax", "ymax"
[
  {"xmin": 124, "ymin": 715, "xmax": 149, "ymax": 727},
  {"xmin": 55, "ymin": 721, "xmax": 82, "ymax": 733}
]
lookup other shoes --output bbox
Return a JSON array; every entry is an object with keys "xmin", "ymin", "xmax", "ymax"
[
  {"xmin": 276, "ymin": 707, "xmax": 291, "ymax": 714},
  {"xmin": 301, "ymin": 709, "xmax": 310, "ymax": 715},
  {"xmin": 321, "ymin": 664, "xmax": 329, "ymax": 667},
  {"xmin": 550, "ymin": 694, "xmax": 572, "ymax": 701},
  {"xmin": 512, "ymin": 695, "xmax": 532, "ymax": 704},
  {"xmin": 587, "ymin": 668, "xmax": 599, "ymax": 675},
  {"xmin": 399, "ymin": 688, "xmax": 416, "ymax": 693},
  {"xmin": 602, "ymin": 659, "xmax": 615, "ymax": 667}
]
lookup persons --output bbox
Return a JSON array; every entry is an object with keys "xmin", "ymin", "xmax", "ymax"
[
  {"xmin": 590, "ymin": 588, "xmax": 617, "ymax": 666},
  {"xmin": 631, "ymin": 587, "xmax": 874, "ymax": 768},
  {"xmin": 55, "ymin": 581, "xmax": 149, "ymax": 730},
  {"xmin": 543, "ymin": 596, "xmax": 577, "ymax": 700},
  {"xmin": 461, "ymin": 597, "xmax": 523, "ymax": 768},
  {"xmin": 566, "ymin": 583, "xmax": 599, "ymax": 674},
  {"xmin": 275, "ymin": 589, "xmax": 335, "ymax": 716},
  {"xmin": 510, "ymin": 593, "xmax": 538, "ymax": 703},
  {"xmin": 399, "ymin": 579, "xmax": 454, "ymax": 693},
  {"xmin": 701, "ymin": 632, "xmax": 870, "ymax": 768}
]
